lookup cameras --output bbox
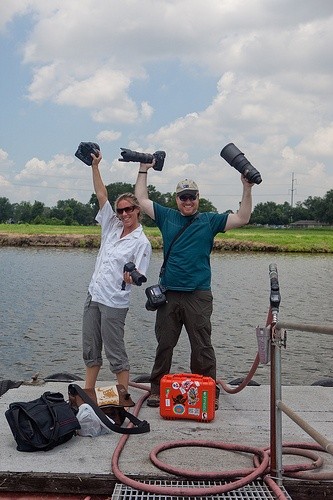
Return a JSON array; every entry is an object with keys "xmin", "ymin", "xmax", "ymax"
[
  {"xmin": 145, "ymin": 285, "xmax": 166, "ymax": 311},
  {"xmin": 74, "ymin": 142, "xmax": 100, "ymax": 166},
  {"xmin": 124, "ymin": 261, "xmax": 147, "ymax": 286},
  {"xmin": 119, "ymin": 147, "xmax": 165, "ymax": 170}
]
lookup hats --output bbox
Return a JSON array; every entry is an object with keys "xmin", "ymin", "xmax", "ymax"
[{"xmin": 176, "ymin": 179, "xmax": 199, "ymax": 193}]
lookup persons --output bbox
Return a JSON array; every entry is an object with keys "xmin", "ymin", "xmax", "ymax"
[
  {"xmin": 134, "ymin": 159, "xmax": 254, "ymax": 411},
  {"xmin": 82, "ymin": 149, "xmax": 152, "ymax": 407}
]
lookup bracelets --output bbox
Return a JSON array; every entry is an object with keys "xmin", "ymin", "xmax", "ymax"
[{"xmin": 139, "ymin": 171, "xmax": 147, "ymax": 175}]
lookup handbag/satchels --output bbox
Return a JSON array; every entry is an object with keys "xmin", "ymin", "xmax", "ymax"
[
  {"xmin": 5, "ymin": 391, "xmax": 81, "ymax": 452},
  {"xmin": 68, "ymin": 383, "xmax": 150, "ymax": 434}
]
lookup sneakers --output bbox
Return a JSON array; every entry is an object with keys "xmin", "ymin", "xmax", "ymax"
[
  {"xmin": 147, "ymin": 390, "xmax": 160, "ymax": 407},
  {"xmin": 215, "ymin": 396, "xmax": 219, "ymax": 410}
]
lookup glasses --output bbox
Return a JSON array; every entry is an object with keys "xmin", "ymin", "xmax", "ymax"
[
  {"xmin": 178, "ymin": 194, "xmax": 197, "ymax": 201},
  {"xmin": 117, "ymin": 205, "xmax": 137, "ymax": 214}
]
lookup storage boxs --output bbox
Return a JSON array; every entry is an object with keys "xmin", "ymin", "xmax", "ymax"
[{"xmin": 159, "ymin": 373, "xmax": 216, "ymax": 422}]
